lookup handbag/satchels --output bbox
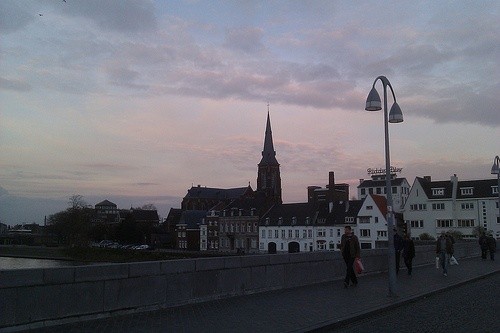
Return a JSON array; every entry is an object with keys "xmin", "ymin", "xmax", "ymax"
[
  {"xmin": 354, "ymin": 260, "xmax": 364, "ymax": 272},
  {"xmin": 436, "ymin": 256, "xmax": 439, "ymax": 268},
  {"xmin": 449, "ymin": 255, "xmax": 458, "ymax": 266}
]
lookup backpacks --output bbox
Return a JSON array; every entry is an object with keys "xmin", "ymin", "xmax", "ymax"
[{"xmin": 482, "ymin": 238, "xmax": 487, "ymax": 246}]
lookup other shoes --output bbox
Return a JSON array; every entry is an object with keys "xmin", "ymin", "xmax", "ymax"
[
  {"xmin": 408, "ymin": 269, "xmax": 412, "ymax": 274},
  {"xmin": 490, "ymin": 258, "xmax": 495, "ymax": 260},
  {"xmin": 443, "ymin": 272, "xmax": 448, "ymax": 276},
  {"xmin": 344, "ymin": 280, "xmax": 349, "ymax": 287},
  {"xmin": 481, "ymin": 254, "xmax": 487, "ymax": 257},
  {"xmin": 351, "ymin": 282, "xmax": 358, "ymax": 287}
]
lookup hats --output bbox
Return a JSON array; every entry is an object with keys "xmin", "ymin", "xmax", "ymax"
[{"xmin": 440, "ymin": 230, "xmax": 448, "ymax": 236}]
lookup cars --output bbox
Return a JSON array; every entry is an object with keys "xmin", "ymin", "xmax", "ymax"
[{"xmin": 90, "ymin": 240, "xmax": 151, "ymax": 250}]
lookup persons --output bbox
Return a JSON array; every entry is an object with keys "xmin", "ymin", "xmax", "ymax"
[
  {"xmin": 340, "ymin": 225, "xmax": 361, "ymax": 288},
  {"xmin": 445, "ymin": 231, "xmax": 454, "ymax": 267},
  {"xmin": 392, "ymin": 228, "xmax": 415, "ymax": 276},
  {"xmin": 435, "ymin": 230, "xmax": 453, "ymax": 277},
  {"xmin": 478, "ymin": 232, "xmax": 490, "ymax": 259},
  {"xmin": 488, "ymin": 234, "xmax": 496, "ymax": 261}
]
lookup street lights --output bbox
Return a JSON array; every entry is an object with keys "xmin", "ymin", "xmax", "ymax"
[
  {"xmin": 491, "ymin": 156, "xmax": 500, "ymax": 219},
  {"xmin": 364, "ymin": 76, "xmax": 405, "ymax": 297}
]
